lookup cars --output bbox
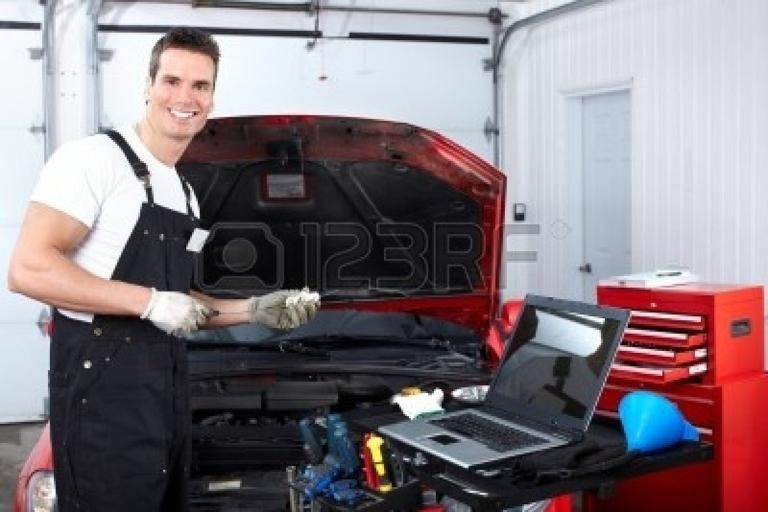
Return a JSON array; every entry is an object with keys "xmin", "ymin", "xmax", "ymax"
[{"xmin": 13, "ymin": 114, "xmax": 526, "ymax": 512}]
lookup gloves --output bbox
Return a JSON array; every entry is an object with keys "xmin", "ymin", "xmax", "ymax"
[
  {"xmin": 139, "ymin": 287, "xmax": 214, "ymax": 337},
  {"xmin": 249, "ymin": 287, "xmax": 322, "ymax": 330}
]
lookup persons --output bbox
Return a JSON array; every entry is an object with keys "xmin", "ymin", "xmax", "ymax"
[{"xmin": 8, "ymin": 26, "xmax": 322, "ymax": 512}]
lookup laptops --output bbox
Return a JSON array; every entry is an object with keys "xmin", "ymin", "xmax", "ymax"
[{"xmin": 378, "ymin": 291, "xmax": 633, "ymax": 470}]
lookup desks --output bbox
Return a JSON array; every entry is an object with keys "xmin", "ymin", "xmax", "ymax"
[{"xmin": 315, "ymin": 404, "xmax": 713, "ymax": 512}]
